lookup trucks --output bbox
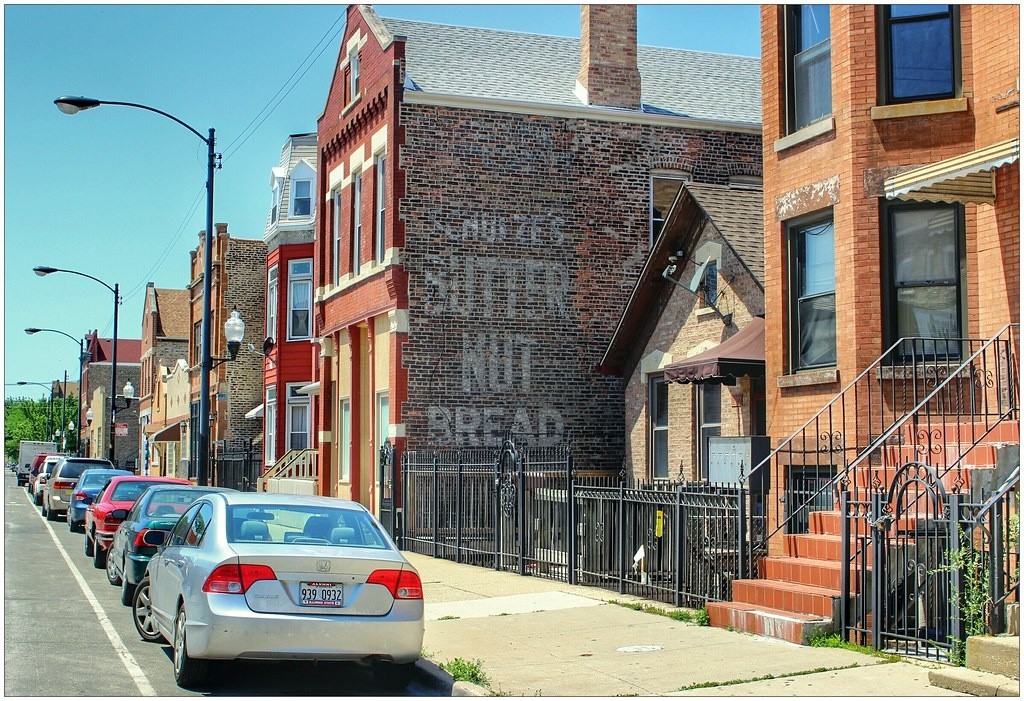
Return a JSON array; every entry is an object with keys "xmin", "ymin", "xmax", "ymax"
[{"xmin": 16, "ymin": 441, "xmax": 57, "ymax": 488}]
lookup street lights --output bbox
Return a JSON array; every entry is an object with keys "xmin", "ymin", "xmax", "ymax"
[
  {"xmin": 53, "ymin": 94, "xmax": 248, "ymax": 485},
  {"xmin": 32, "ymin": 265, "xmax": 126, "ymax": 466},
  {"xmin": 15, "ymin": 379, "xmax": 56, "ymax": 439},
  {"xmin": 22, "ymin": 326, "xmax": 88, "ymax": 458}
]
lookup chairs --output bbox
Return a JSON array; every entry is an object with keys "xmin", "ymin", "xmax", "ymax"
[
  {"xmin": 155, "ymin": 504, "xmax": 176, "ymax": 517},
  {"xmin": 331, "ymin": 526, "xmax": 356, "ymax": 544},
  {"xmin": 235, "ymin": 519, "xmax": 271, "ymax": 541},
  {"xmin": 303, "ymin": 515, "xmax": 340, "ymax": 541}
]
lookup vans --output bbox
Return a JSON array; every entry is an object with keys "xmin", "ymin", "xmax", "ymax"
[
  {"xmin": 41, "ymin": 457, "xmax": 116, "ymax": 520},
  {"xmin": 24, "ymin": 453, "xmax": 67, "ymax": 494}
]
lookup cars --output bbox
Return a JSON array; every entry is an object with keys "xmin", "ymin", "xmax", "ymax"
[
  {"xmin": 30, "ymin": 456, "xmax": 65, "ymax": 506},
  {"xmin": 6, "ymin": 461, "xmax": 19, "ymax": 473},
  {"xmin": 67, "ymin": 469, "xmax": 135, "ymax": 533},
  {"xmin": 132, "ymin": 492, "xmax": 426, "ymax": 689},
  {"xmin": 104, "ymin": 484, "xmax": 245, "ymax": 607},
  {"xmin": 81, "ymin": 477, "xmax": 195, "ymax": 568}
]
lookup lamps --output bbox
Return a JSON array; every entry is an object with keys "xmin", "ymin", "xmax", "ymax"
[
  {"xmin": 209, "ymin": 411, "xmax": 218, "ymax": 427},
  {"xmin": 180, "ymin": 417, "xmax": 191, "ymax": 433}
]
